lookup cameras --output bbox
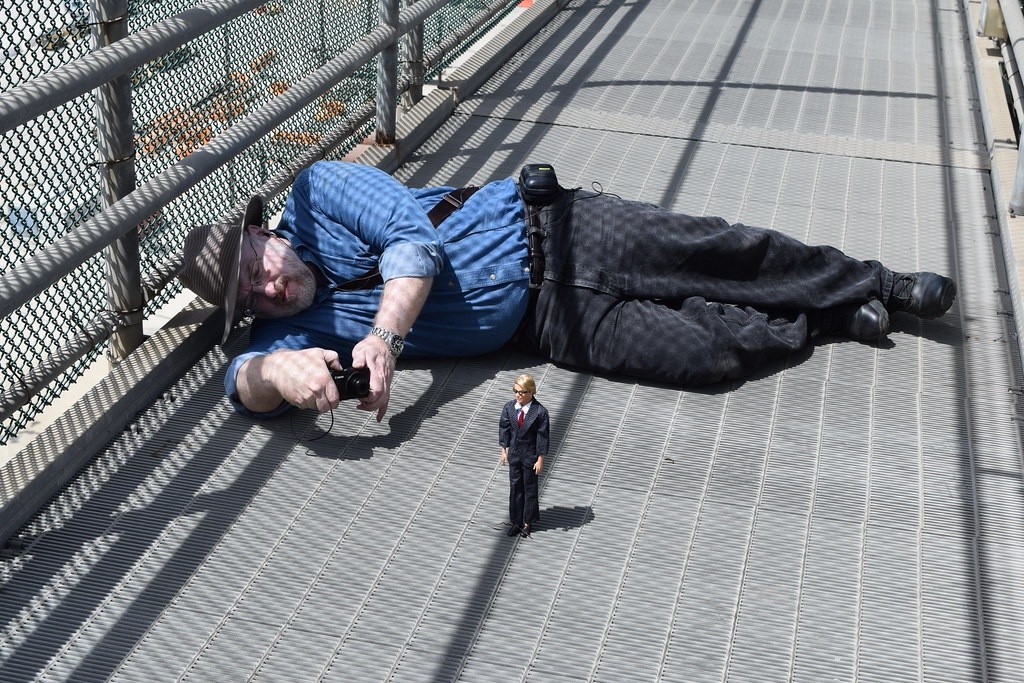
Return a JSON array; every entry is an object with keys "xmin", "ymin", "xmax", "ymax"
[{"xmin": 330, "ymin": 366, "xmax": 370, "ymax": 401}]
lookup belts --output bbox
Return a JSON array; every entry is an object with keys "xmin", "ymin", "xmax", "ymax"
[{"xmin": 506, "ymin": 182, "xmax": 544, "ymax": 348}]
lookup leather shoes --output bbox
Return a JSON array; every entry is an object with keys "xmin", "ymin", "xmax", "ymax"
[
  {"xmin": 833, "ymin": 295, "xmax": 890, "ymax": 341},
  {"xmin": 888, "ymin": 271, "xmax": 957, "ymax": 320}
]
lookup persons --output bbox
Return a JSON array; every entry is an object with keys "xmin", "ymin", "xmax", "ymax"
[
  {"xmin": 178, "ymin": 161, "xmax": 957, "ymax": 424},
  {"xmin": 498, "ymin": 373, "xmax": 550, "ymax": 537}
]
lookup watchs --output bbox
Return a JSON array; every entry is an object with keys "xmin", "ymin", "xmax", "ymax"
[{"xmin": 369, "ymin": 327, "xmax": 405, "ymax": 357}]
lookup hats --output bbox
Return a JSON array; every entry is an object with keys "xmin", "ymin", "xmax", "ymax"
[{"xmin": 178, "ymin": 195, "xmax": 263, "ymax": 351}]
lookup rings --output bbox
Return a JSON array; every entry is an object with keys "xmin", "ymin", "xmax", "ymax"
[{"xmin": 385, "ymin": 393, "xmax": 390, "ymax": 401}]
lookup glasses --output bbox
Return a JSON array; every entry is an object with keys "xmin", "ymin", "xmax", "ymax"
[{"xmin": 239, "ymin": 230, "xmax": 268, "ymax": 318}]
